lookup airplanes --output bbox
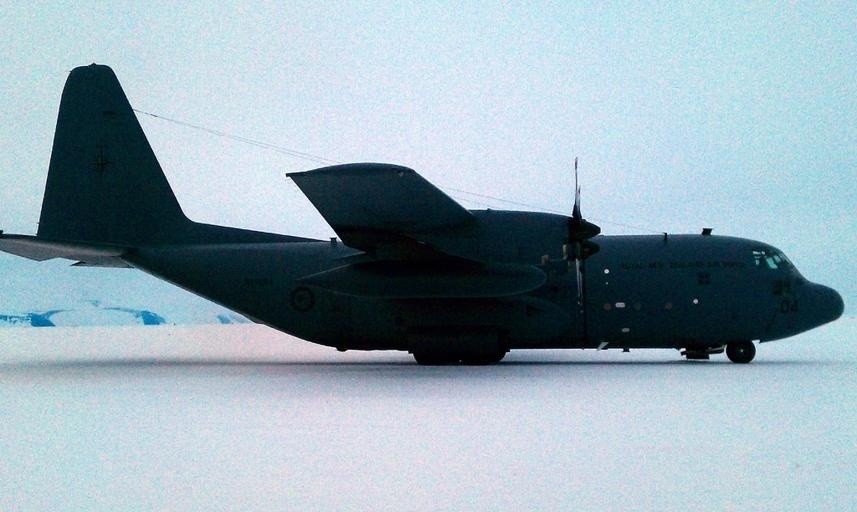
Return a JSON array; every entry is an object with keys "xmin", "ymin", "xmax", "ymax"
[{"xmin": 0, "ymin": 63, "xmax": 844, "ymax": 366}]
